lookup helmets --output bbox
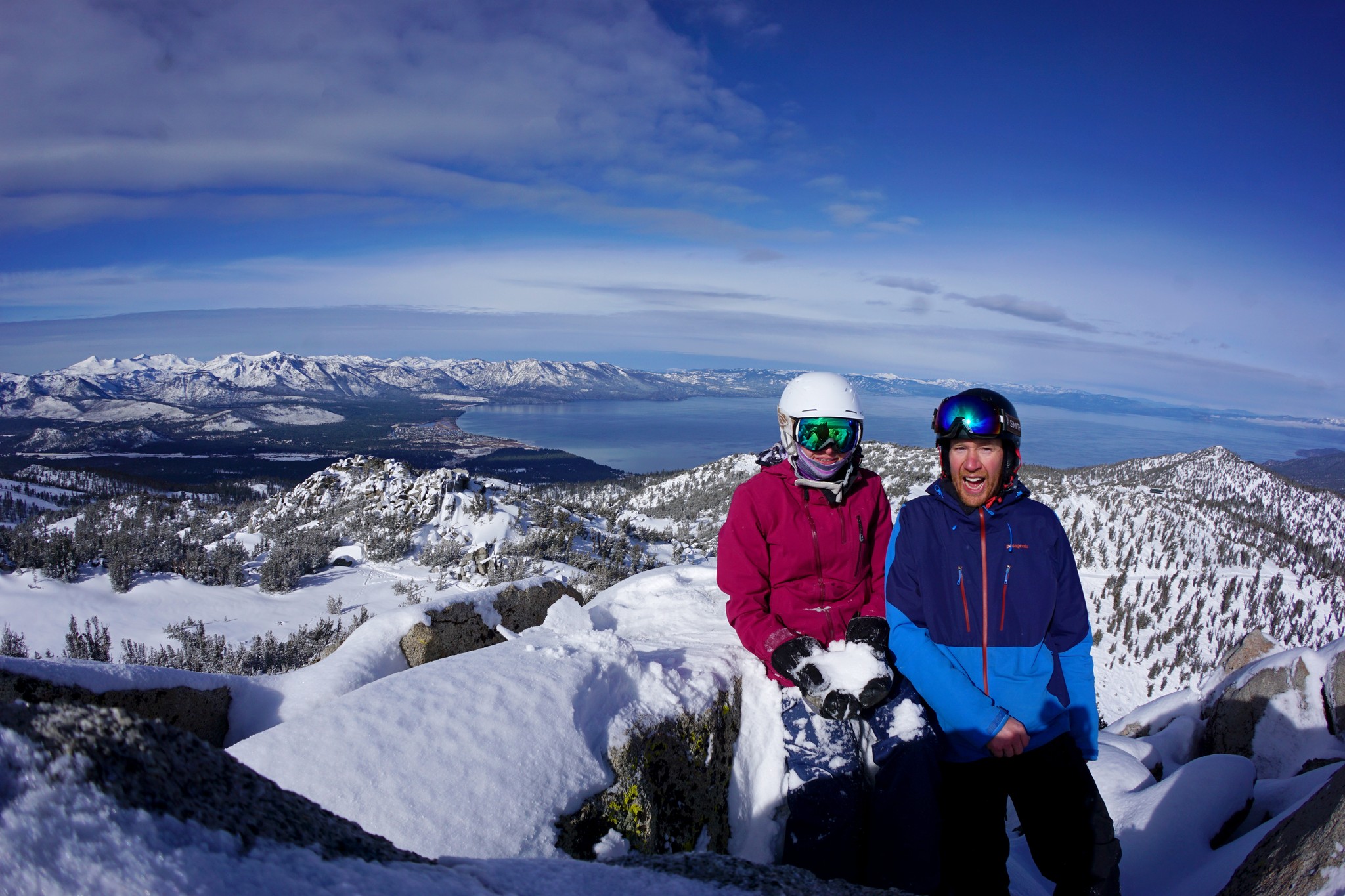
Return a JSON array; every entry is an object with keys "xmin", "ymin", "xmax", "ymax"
[
  {"xmin": 928, "ymin": 387, "xmax": 1021, "ymax": 489},
  {"xmin": 777, "ymin": 372, "xmax": 864, "ymax": 458}
]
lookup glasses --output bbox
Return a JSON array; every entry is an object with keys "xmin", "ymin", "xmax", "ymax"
[
  {"xmin": 930, "ymin": 396, "xmax": 1021, "ymax": 438},
  {"xmin": 774, "ymin": 406, "xmax": 860, "ymax": 452}
]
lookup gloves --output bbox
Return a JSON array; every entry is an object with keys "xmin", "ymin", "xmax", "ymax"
[
  {"xmin": 842, "ymin": 615, "xmax": 897, "ymax": 709},
  {"xmin": 770, "ymin": 634, "xmax": 867, "ymax": 724}
]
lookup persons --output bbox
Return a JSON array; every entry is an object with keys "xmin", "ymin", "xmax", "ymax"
[
  {"xmin": 718, "ymin": 372, "xmax": 943, "ymax": 894},
  {"xmin": 884, "ymin": 387, "xmax": 1121, "ymax": 896}
]
